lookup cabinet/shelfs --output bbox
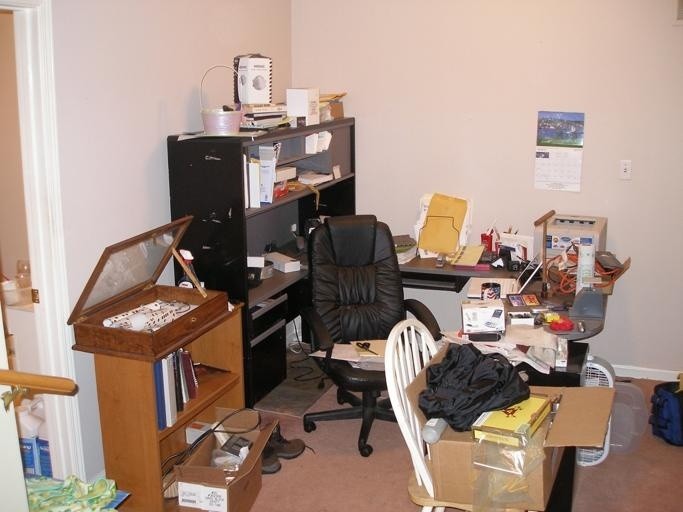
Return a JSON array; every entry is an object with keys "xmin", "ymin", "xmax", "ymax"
[
  {"xmin": 85, "ymin": 302, "xmax": 244, "ymax": 511},
  {"xmin": 168, "ymin": 117, "xmax": 360, "ymax": 409}
]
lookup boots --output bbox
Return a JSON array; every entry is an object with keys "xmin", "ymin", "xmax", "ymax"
[
  {"xmin": 270, "ymin": 424, "xmax": 305, "ymax": 458},
  {"xmin": 261, "ymin": 444, "xmax": 281, "ymax": 473}
]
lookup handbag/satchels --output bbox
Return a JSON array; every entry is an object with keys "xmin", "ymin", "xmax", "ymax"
[
  {"xmin": 25, "ymin": 476, "xmax": 119, "ymax": 512},
  {"xmin": 418, "ymin": 342, "xmax": 530, "ymax": 432},
  {"xmin": 649, "ymin": 382, "xmax": 683, "ymax": 446}
]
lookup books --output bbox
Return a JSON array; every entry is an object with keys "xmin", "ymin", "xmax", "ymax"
[
  {"xmin": 392, "ymin": 233, "xmax": 418, "ymax": 266},
  {"xmin": 450, "ymin": 244, "xmax": 485, "ymax": 267},
  {"xmin": 307, "ymin": 336, "xmax": 387, "ymax": 372},
  {"xmin": 240, "ymin": 92, "xmax": 346, "ymax": 133},
  {"xmin": 154, "ymin": 346, "xmax": 199, "ymax": 430}
]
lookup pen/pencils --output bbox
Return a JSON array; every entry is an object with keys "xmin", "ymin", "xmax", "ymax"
[{"xmin": 508, "ymin": 224, "xmax": 519, "ymax": 234}]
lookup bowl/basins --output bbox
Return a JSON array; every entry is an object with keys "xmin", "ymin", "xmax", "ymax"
[{"xmin": 2, "ymin": 279, "xmax": 34, "ymax": 308}]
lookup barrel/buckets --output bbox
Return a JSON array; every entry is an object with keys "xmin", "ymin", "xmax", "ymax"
[{"xmin": 197, "ymin": 63, "xmax": 245, "ymax": 133}]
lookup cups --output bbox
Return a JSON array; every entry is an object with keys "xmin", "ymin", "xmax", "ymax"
[
  {"xmin": 261, "ymin": 262, "xmax": 274, "ymax": 280},
  {"xmin": 480, "ymin": 283, "xmax": 501, "ymax": 301}
]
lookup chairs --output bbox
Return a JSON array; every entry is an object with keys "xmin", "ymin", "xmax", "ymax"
[
  {"xmin": 381, "ymin": 316, "xmax": 441, "ymax": 511},
  {"xmin": 302, "ymin": 212, "xmax": 443, "ymax": 461}
]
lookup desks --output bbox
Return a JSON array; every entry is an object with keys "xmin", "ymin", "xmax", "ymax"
[{"xmin": 399, "ymin": 234, "xmax": 611, "ymax": 342}]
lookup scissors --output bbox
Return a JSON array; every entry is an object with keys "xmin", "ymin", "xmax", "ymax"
[{"xmin": 356, "ymin": 342, "xmax": 378, "ymax": 355}]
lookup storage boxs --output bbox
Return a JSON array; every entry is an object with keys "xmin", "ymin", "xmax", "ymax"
[
  {"xmin": 401, "ymin": 341, "xmax": 616, "ymax": 507},
  {"xmin": 167, "ymin": 408, "xmax": 278, "ymax": 511},
  {"xmin": 65, "ymin": 215, "xmax": 232, "ymax": 355}
]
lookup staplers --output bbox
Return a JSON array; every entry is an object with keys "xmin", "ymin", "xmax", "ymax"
[{"xmin": 435, "ymin": 252, "xmax": 446, "ymax": 267}]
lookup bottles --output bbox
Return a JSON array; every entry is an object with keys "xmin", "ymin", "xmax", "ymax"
[
  {"xmin": 421, "ymin": 417, "xmax": 447, "ymax": 444},
  {"xmin": 480, "ymin": 234, "xmax": 492, "ymax": 252}
]
